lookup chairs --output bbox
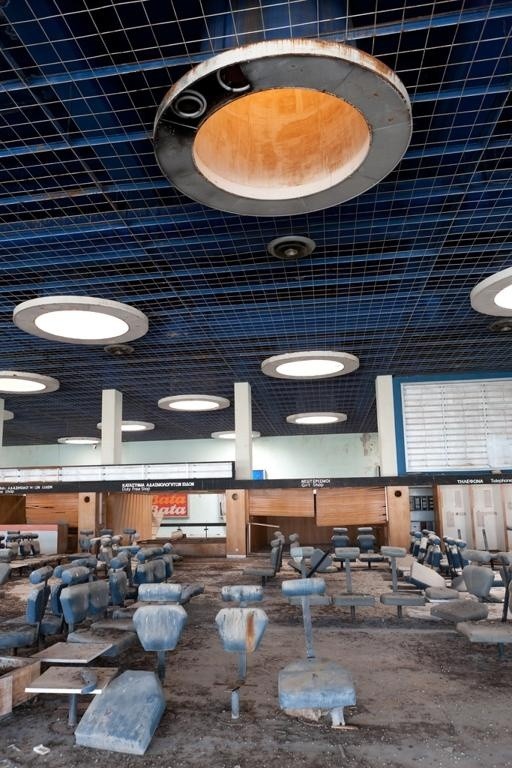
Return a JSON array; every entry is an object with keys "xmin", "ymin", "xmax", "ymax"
[{"xmin": 0, "ymin": 528, "xmax": 512, "ymax": 755}]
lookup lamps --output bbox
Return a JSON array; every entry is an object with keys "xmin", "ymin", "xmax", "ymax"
[
  {"xmin": 153, "ymin": 0, "xmax": 414, "ymax": 219},
  {"xmin": 0, "ymin": 372, "xmax": 59, "ymax": 397},
  {"xmin": 261, "ymin": 350, "xmax": 360, "ymax": 382},
  {"xmin": 469, "ymin": 266, "xmax": 512, "ymax": 327},
  {"xmin": 58, "ymin": 394, "xmax": 260, "ymax": 444},
  {"xmin": 13, "ymin": 293, "xmax": 149, "ymax": 347},
  {"xmin": 285, "ymin": 411, "xmax": 347, "ymax": 426}
]
